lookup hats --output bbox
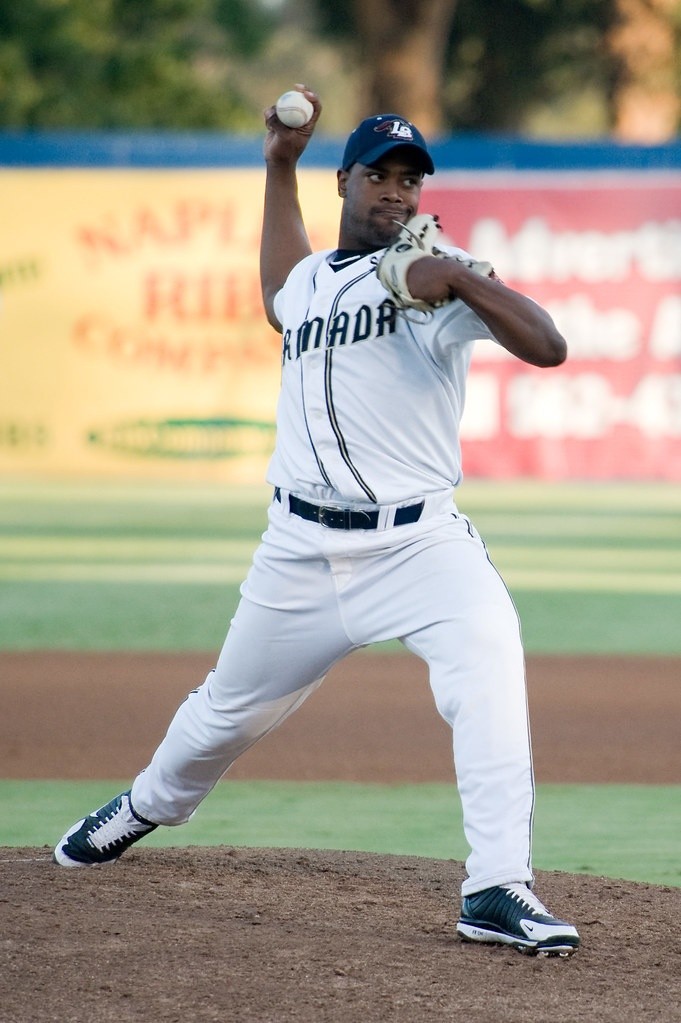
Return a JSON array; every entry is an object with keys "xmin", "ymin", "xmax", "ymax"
[{"xmin": 342, "ymin": 114, "xmax": 434, "ymax": 175}]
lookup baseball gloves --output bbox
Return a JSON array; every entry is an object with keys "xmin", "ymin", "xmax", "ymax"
[{"xmin": 378, "ymin": 211, "xmax": 495, "ymax": 309}]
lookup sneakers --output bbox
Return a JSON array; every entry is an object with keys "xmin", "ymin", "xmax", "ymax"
[
  {"xmin": 53, "ymin": 788, "xmax": 158, "ymax": 867},
  {"xmin": 456, "ymin": 882, "xmax": 580, "ymax": 957}
]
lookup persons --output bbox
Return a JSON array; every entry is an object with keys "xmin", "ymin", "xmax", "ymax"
[{"xmin": 53, "ymin": 82, "xmax": 580, "ymax": 958}]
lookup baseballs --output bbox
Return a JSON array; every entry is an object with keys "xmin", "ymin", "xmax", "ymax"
[{"xmin": 276, "ymin": 89, "xmax": 314, "ymax": 127}]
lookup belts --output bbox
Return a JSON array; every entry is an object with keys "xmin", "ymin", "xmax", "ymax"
[{"xmin": 274, "ymin": 486, "xmax": 423, "ymax": 531}]
